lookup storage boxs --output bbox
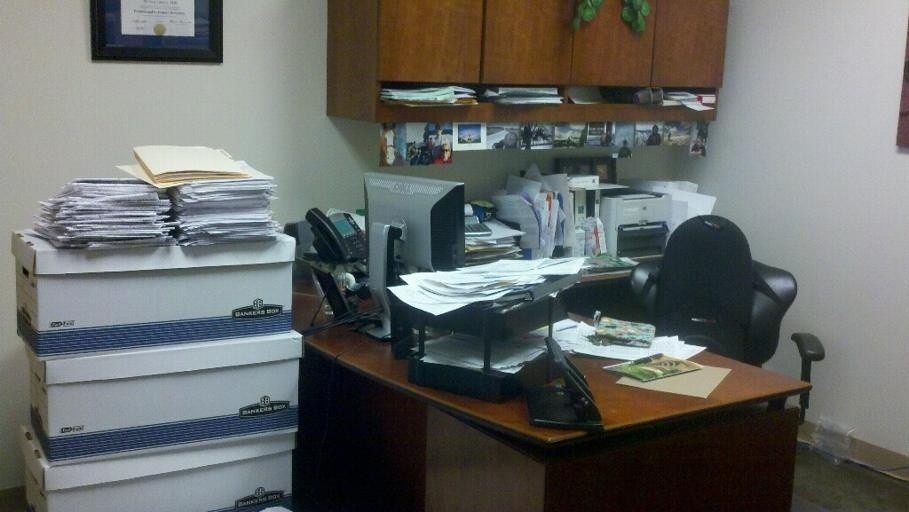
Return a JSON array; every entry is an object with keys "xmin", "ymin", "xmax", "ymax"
[
  {"xmin": 11, "ymin": 230, "xmax": 295, "ymax": 359},
  {"xmin": 22, "ymin": 427, "xmax": 298, "ymax": 512},
  {"xmin": 24, "ymin": 330, "xmax": 304, "ymax": 466}
]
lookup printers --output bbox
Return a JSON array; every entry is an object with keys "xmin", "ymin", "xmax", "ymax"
[{"xmin": 600, "ymin": 189, "xmax": 672, "ymax": 265}]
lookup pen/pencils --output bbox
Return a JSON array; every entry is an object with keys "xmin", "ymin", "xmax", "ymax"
[{"xmin": 628, "ymin": 352, "xmax": 664, "ymax": 367}]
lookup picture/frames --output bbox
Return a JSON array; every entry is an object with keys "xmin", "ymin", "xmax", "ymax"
[{"xmin": 90, "ymin": 0, "xmax": 224, "ymax": 64}]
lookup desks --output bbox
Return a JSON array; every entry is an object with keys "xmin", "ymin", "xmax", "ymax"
[{"xmin": 297, "ymin": 254, "xmax": 811, "ymax": 512}]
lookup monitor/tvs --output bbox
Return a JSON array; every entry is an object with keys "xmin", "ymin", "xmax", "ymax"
[{"xmin": 361, "ymin": 170, "xmax": 465, "ymax": 343}]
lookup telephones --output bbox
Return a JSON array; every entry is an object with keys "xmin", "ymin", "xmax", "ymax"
[{"xmin": 304, "ymin": 206, "xmax": 368, "ymax": 265}]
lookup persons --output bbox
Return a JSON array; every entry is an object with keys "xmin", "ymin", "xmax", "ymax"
[
  {"xmin": 647, "ymin": 125, "xmax": 660, "ymax": 146},
  {"xmin": 618, "ymin": 139, "xmax": 631, "ymax": 159},
  {"xmin": 692, "ymin": 120, "xmax": 708, "ymax": 156},
  {"xmin": 382, "ymin": 122, "xmax": 453, "ymax": 166}
]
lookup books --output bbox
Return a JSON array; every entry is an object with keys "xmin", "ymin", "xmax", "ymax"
[
  {"xmin": 596, "ymin": 317, "xmax": 656, "ymax": 348},
  {"xmin": 579, "ymin": 254, "xmax": 633, "ymax": 272},
  {"xmin": 133, "ymin": 146, "xmax": 242, "ymax": 184},
  {"xmin": 381, "ymin": 85, "xmax": 564, "ymax": 107}
]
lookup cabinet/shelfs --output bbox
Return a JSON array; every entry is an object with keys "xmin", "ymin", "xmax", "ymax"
[
  {"xmin": 569, "ymin": 0, "xmax": 730, "ymax": 122},
  {"xmin": 328, "ymin": 0, "xmax": 572, "ymax": 123}
]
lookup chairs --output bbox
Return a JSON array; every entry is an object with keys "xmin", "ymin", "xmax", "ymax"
[{"xmin": 627, "ymin": 215, "xmax": 825, "ymax": 425}]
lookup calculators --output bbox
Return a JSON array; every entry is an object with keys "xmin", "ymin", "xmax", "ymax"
[{"xmin": 463, "ymin": 214, "xmax": 494, "ymax": 237}]
